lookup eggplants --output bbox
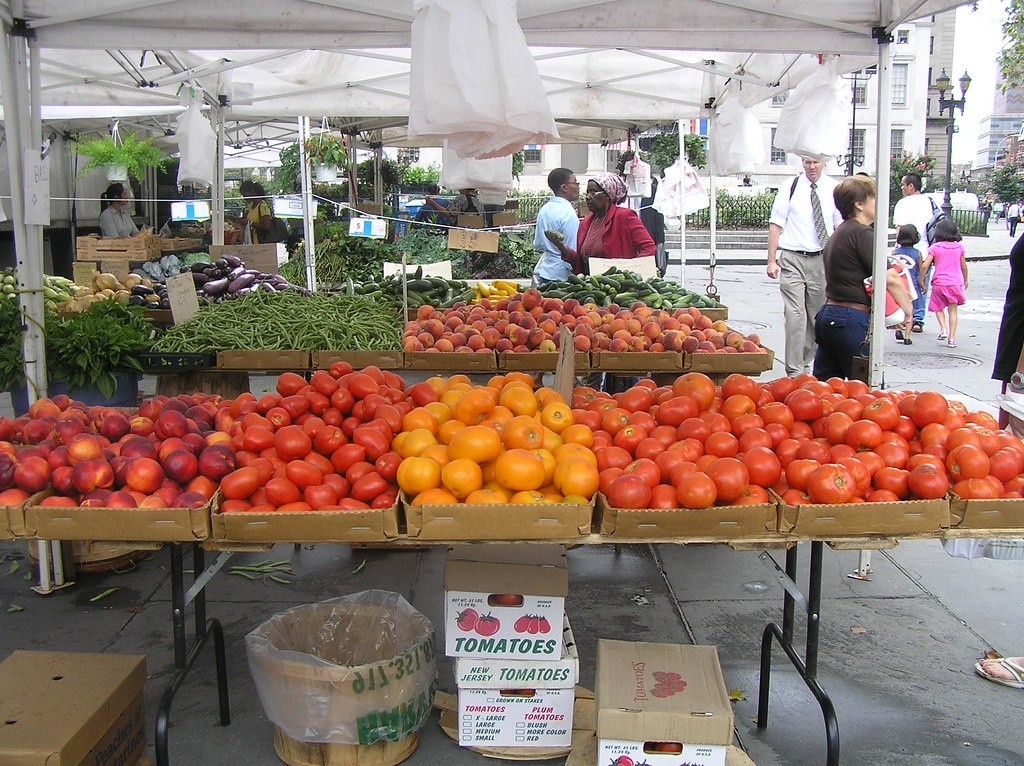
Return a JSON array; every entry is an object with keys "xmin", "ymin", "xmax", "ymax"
[{"xmin": 130, "ymin": 254, "xmax": 313, "ymax": 308}]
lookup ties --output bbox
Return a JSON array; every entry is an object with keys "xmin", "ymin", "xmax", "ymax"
[{"xmin": 810, "ymin": 183, "xmax": 830, "ymax": 250}]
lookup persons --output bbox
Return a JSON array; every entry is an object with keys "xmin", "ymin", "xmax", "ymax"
[
  {"xmin": 766, "ymin": 158, "xmax": 845, "ymax": 378},
  {"xmin": 414, "ymin": 187, "xmax": 487, "ymax": 231},
  {"xmin": 891, "ymin": 224, "xmax": 927, "ymax": 344},
  {"xmin": 234, "ymin": 180, "xmax": 271, "ymax": 244},
  {"xmin": 533, "ymin": 168, "xmax": 581, "ymax": 285},
  {"xmin": 893, "ymin": 173, "xmax": 945, "ymax": 333},
  {"xmin": 983, "ymin": 199, "xmax": 1024, "ymax": 237},
  {"xmin": 920, "ymin": 220, "xmax": 969, "ymax": 347},
  {"xmin": 544, "ymin": 172, "xmax": 657, "ymax": 275},
  {"xmin": 853, "ymin": 172, "xmax": 890, "ymax": 230},
  {"xmin": 975, "ymin": 232, "xmax": 1024, "ymax": 696},
  {"xmin": 98, "ymin": 183, "xmax": 140, "ymax": 240},
  {"xmin": 812, "ymin": 174, "xmax": 913, "ymax": 389},
  {"xmin": 640, "ymin": 173, "xmax": 669, "ymax": 277}
]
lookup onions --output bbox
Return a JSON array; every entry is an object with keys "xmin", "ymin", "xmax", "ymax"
[{"xmin": 62, "ymin": 267, "xmax": 142, "ymax": 313}]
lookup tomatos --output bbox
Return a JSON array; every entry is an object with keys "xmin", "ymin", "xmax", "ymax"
[
  {"xmin": 499, "ymin": 689, "xmax": 535, "ymax": 696},
  {"xmin": 645, "ymin": 743, "xmax": 681, "ymax": 752},
  {"xmin": 489, "ymin": 593, "xmax": 520, "ymax": 605},
  {"xmin": 221, "ymin": 361, "xmax": 599, "ymax": 512},
  {"xmin": 570, "ymin": 372, "xmax": 1024, "ymax": 508}
]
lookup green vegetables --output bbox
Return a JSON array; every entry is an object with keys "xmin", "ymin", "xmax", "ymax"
[
  {"xmin": 0, "ymin": 294, "xmax": 162, "ymax": 398},
  {"xmin": 279, "ymin": 230, "xmax": 542, "ymax": 285}
]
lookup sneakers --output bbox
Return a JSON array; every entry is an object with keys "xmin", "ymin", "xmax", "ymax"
[
  {"xmin": 937, "ymin": 329, "xmax": 948, "ymax": 340},
  {"xmin": 947, "ymin": 339, "xmax": 956, "ymax": 347}
]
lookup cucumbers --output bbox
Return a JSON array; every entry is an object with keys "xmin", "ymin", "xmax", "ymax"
[{"xmin": 537, "ymin": 266, "xmax": 716, "ymax": 310}]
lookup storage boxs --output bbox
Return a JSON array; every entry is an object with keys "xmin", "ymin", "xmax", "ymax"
[
  {"xmin": 0, "ymin": 648, "xmax": 150, "ymax": 766},
  {"xmin": 443, "ymin": 544, "xmax": 734, "ymax": 766},
  {"xmin": 75, "ymin": 232, "xmax": 202, "ymax": 292},
  {"xmin": 0, "ymin": 304, "xmax": 1024, "ymax": 541},
  {"xmin": 209, "ymin": 242, "xmax": 289, "ymax": 276},
  {"xmin": 457, "ymin": 215, "xmax": 483, "ymax": 229},
  {"xmin": 492, "ymin": 212, "xmax": 516, "ymax": 226},
  {"xmin": 578, "ymin": 200, "xmax": 592, "ymax": 217}
]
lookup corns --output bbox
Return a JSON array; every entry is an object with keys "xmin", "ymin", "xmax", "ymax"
[{"xmin": 0, "ymin": 273, "xmax": 80, "ymax": 309}]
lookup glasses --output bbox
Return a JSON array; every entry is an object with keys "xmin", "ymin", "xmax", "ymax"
[
  {"xmin": 558, "ymin": 181, "xmax": 580, "ymax": 188},
  {"xmin": 583, "ymin": 190, "xmax": 605, "ymax": 198}
]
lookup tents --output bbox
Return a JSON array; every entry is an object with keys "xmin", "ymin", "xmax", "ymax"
[{"xmin": 0, "ymin": 0, "xmax": 989, "ymax": 594}]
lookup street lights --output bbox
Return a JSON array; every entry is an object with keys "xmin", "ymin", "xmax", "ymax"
[{"xmin": 934, "ymin": 66, "xmax": 973, "ymax": 220}]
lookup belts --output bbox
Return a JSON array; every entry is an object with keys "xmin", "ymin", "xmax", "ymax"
[
  {"xmin": 824, "ymin": 298, "xmax": 870, "ymax": 313},
  {"xmin": 796, "ymin": 250, "xmax": 823, "ymax": 256}
]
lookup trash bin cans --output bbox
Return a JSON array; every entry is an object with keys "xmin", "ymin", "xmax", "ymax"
[{"xmin": 256, "ymin": 607, "xmax": 429, "ymax": 766}]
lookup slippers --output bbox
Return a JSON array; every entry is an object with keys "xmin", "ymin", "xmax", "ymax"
[
  {"xmin": 975, "ymin": 656, "xmax": 1024, "ymax": 688},
  {"xmin": 913, "ymin": 324, "xmax": 923, "ymax": 333}
]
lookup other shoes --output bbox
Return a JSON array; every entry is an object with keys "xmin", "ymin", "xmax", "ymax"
[
  {"xmin": 904, "ymin": 339, "xmax": 912, "ymax": 345},
  {"xmin": 895, "ymin": 330, "xmax": 903, "ymax": 340}
]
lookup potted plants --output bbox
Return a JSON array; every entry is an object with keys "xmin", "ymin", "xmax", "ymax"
[{"xmin": 68, "ymin": 131, "xmax": 167, "ymax": 182}]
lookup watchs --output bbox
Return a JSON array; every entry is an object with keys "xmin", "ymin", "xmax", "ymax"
[{"xmin": 1010, "ymin": 372, "xmax": 1024, "ymax": 391}]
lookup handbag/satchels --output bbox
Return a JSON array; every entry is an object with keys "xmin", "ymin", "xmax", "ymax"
[
  {"xmin": 1017, "ymin": 214, "xmax": 1021, "ymax": 222},
  {"xmin": 926, "ymin": 197, "xmax": 948, "ymax": 263},
  {"xmin": 257, "ymin": 202, "xmax": 291, "ymax": 243}
]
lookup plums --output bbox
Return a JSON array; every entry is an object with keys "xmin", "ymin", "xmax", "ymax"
[{"xmin": 0, "ymin": 393, "xmax": 256, "ymax": 508}]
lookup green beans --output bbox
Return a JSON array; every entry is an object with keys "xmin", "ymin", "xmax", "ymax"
[
  {"xmin": 148, "ymin": 285, "xmax": 404, "ymax": 366},
  {"xmin": 231, "ymin": 559, "xmax": 298, "ymax": 585}
]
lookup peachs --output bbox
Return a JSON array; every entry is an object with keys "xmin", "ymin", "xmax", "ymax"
[{"xmin": 403, "ymin": 289, "xmax": 768, "ymax": 354}]
